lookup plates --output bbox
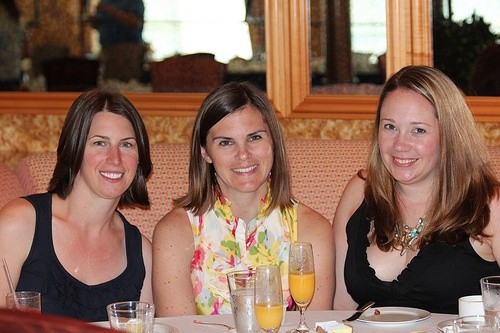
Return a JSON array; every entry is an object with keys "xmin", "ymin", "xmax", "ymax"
[
  {"xmin": 357, "ymin": 306, "xmax": 431, "ymax": 324},
  {"xmin": 155, "ymin": 322, "xmax": 179, "ymax": 333}
]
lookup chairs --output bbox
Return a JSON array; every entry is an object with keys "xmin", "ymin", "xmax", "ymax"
[{"xmin": 149, "ymin": 53, "xmax": 219, "ymax": 93}]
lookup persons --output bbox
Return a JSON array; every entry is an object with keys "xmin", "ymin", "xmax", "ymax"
[
  {"xmin": 0, "ymin": 89, "xmax": 155, "ymax": 322},
  {"xmin": 0, "ymin": 0, "xmax": 78, "ymax": 92},
  {"xmin": 151, "ymin": 82, "xmax": 335, "ymax": 319},
  {"xmin": 88, "ymin": 0, "xmax": 145, "ymax": 87},
  {"xmin": 332, "ymin": 64, "xmax": 500, "ymax": 316}
]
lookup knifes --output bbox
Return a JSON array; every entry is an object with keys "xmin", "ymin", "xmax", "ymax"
[{"xmin": 342, "ymin": 301, "xmax": 374, "ymax": 322}]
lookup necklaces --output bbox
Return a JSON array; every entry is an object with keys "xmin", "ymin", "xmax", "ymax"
[{"xmin": 391, "ymin": 215, "xmax": 428, "ymax": 255}]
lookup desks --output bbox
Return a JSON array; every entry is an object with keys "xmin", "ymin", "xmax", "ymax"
[{"xmin": 89, "ymin": 310, "xmax": 460, "ymax": 333}]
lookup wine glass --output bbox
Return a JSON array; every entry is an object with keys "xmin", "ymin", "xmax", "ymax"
[{"xmin": 286, "ymin": 241, "xmax": 318, "ymax": 333}]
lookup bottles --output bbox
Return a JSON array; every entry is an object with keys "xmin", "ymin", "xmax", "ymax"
[{"xmin": 15, "ymin": 56, "xmax": 43, "ymax": 92}]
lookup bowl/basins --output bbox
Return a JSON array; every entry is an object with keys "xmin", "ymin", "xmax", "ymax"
[{"xmin": 437, "ymin": 315, "xmax": 500, "ymax": 333}]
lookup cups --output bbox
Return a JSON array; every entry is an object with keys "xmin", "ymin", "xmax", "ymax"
[
  {"xmin": 254, "ymin": 264, "xmax": 284, "ymax": 333},
  {"xmin": 6, "ymin": 291, "xmax": 42, "ymax": 314},
  {"xmin": 227, "ymin": 269, "xmax": 264, "ymax": 333},
  {"xmin": 106, "ymin": 301, "xmax": 156, "ymax": 333},
  {"xmin": 459, "ymin": 295, "xmax": 483, "ymax": 318},
  {"xmin": 480, "ymin": 274, "xmax": 500, "ymax": 316}
]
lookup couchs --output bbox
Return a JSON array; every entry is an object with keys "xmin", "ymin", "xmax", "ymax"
[{"xmin": 0, "ymin": 138, "xmax": 500, "ymax": 240}]
